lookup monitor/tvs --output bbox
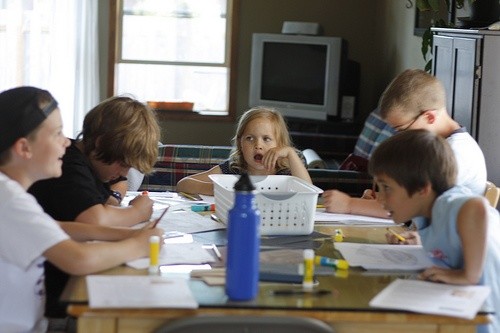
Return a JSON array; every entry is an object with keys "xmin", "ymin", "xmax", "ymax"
[{"xmin": 249, "ymin": 33, "xmax": 342, "ymax": 121}]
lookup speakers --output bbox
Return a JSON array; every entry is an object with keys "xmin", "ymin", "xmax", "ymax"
[
  {"xmin": 341, "ymin": 97, "xmax": 355, "ymax": 122},
  {"xmin": 281, "ymin": 21, "xmax": 324, "ymax": 36}
]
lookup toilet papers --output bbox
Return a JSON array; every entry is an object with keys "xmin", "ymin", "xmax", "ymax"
[{"xmin": 301, "ymin": 148, "xmax": 329, "ymax": 169}]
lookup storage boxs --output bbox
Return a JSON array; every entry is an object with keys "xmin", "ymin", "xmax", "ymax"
[
  {"xmin": 208, "ymin": 174, "xmax": 323, "ymax": 235},
  {"xmin": 148, "ymin": 101, "xmax": 193, "ymax": 111}
]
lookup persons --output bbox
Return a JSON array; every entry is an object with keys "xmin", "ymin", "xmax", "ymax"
[
  {"xmin": 368, "ymin": 129, "xmax": 500, "ymax": 333},
  {"xmin": 0, "ymin": 86, "xmax": 164, "ymax": 333},
  {"xmin": 321, "ymin": 68, "xmax": 487, "ymax": 220},
  {"xmin": 176, "ymin": 106, "xmax": 313, "ymax": 195},
  {"xmin": 28, "ymin": 96, "xmax": 161, "ymax": 319}
]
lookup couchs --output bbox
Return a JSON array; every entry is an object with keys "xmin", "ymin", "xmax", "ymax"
[{"xmin": 149, "ymin": 142, "xmax": 233, "ymax": 185}]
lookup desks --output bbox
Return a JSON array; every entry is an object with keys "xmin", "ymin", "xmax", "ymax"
[
  {"xmin": 57, "ymin": 191, "xmax": 492, "ymax": 332},
  {"xmin": 284, "ymin": 116, "xmax": 363, "ymax": 160}
]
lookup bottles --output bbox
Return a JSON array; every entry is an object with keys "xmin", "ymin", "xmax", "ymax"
[{"xmin": 225, "ymin": 171, "xmax": 260, "ymax": 301}]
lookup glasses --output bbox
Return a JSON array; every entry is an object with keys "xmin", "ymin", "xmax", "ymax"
[{"xmin": 394, "ymin": 109, "xmax": 437, "ymax": 134}]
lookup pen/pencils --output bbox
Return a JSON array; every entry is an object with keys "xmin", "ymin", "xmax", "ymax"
[
  {"xmin": 152, "ymin": 207, "xmax": 170, "ymax": 229},
  {"xmin": 180, "ymin": 191, "xmax": 197, "ymax": 200},
  {"xmin": 372, "ymin": 180, "xmax": 376, "ymax": 199},
  {"xmin": 387, "ymin": 227, "xmax": 405, "ymax": 241},
  {"xmin": 272, "ymin": 290, "xmax": 333, "ymax": 296},
  {"xmin": 213, "ymin": 244, "xmax": 222, "ymax": 261}
]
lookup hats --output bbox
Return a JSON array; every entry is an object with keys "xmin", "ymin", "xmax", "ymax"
[{"xmin": 0, "ymin": 87, "xmax": 57, "ymax": 152}]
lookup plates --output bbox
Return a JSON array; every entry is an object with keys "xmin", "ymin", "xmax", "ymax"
[{"xmin": 147, "ymin": 102, "xmax": 193, "ymax": 111}]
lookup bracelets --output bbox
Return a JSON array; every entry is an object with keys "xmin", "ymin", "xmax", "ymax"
[{"xmin": 112, "ymin": 190, "xmax": 121, "ymax": 200}]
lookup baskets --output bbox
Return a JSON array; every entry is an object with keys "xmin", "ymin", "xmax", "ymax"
[{"xmin": 209, "ymin": 174, "xmax": 324, "ymax": 236}]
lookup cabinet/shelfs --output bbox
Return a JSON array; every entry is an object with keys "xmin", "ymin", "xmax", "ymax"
[{"xmin": 430, "ymin": 27, "xmax": 500, "ymax": 187}]
